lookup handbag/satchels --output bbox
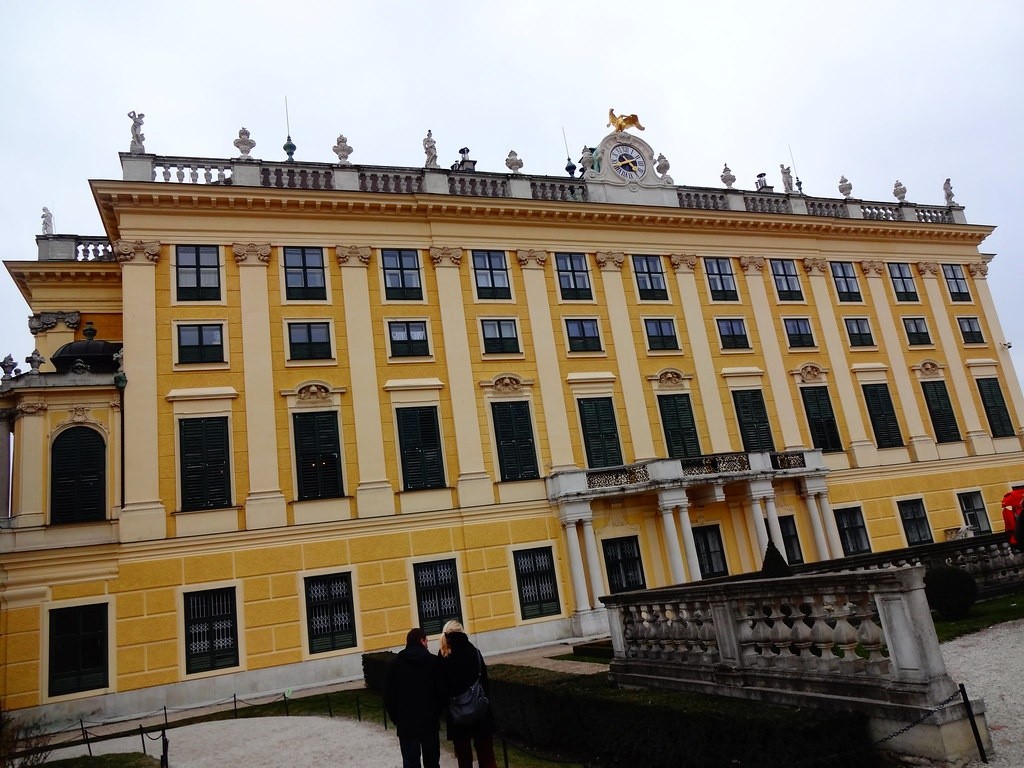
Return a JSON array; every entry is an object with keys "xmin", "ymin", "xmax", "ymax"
[{"xmin": 445, "ymin": 683, "xmax": 490, "ymax": 723}]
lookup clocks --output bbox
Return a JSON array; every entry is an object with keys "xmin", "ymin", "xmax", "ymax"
[{"xmin": 609, "ymin": 144, "xmax": 648, "ymax": 183}]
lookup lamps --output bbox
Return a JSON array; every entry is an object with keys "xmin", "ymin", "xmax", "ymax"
[{"xmin": 1000, "ymin": 341, "xmax": 1013, "ymax": 350}]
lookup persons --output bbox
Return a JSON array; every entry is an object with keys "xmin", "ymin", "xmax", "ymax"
[
  {"xmin": 380, "ymin": 628, "xmax": 448, "ymax": 768},
  {"xmin": 437, "ymin": 621, "xmax": 496, "ymax": 768}
]
